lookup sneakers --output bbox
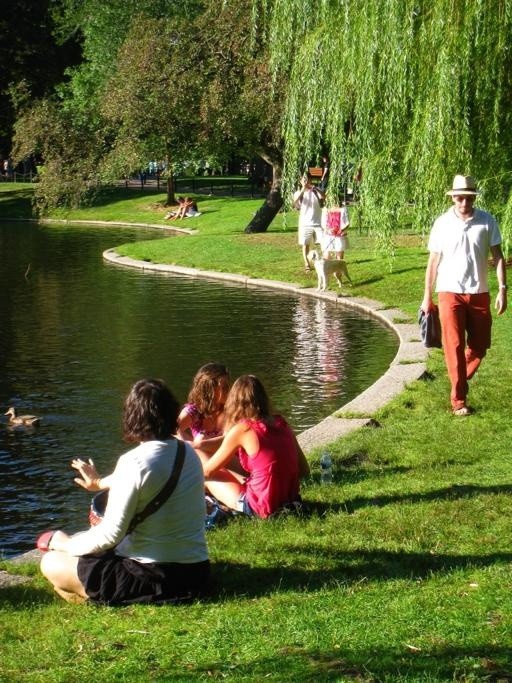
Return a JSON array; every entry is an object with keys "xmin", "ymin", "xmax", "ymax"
[{"xmin": 455, "ymin": 406, "xmax": 471, "ymax": 416}]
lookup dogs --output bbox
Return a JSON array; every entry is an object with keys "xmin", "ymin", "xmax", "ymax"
[{"xmin": 307, "ymin": 250, "xmax": 353, "ymax": 291}]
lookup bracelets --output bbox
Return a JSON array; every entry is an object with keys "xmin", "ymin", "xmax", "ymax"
[{"xmin": 499, "ymin": 284, "xmax": 507, "ymax": 290}]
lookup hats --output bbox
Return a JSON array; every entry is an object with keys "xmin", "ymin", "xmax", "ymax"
[{"xmin": 446, "ymin": 174, "xmax": 480, "ymax": 196}]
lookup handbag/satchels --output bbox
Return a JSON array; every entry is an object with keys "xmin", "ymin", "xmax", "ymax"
[{"xmin": 89, "ymin": 488, "xmax": 133, "ymax": 557}]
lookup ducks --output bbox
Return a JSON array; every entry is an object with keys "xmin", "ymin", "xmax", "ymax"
[{"xmin": 4, "ymin": 407, "xmax": 44, "ymax": 426}]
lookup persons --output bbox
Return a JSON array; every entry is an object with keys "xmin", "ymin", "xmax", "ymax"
[
  {"xmin": 0, "ymin": 155, "xmax": 13, "ymax": 177},
  {"xmin": 175, "ymin": 197, "xmax": 198, "ymax": 222},
  {"xmin": 319, "ymin": 189, "xmax": 349, "ymax": 281},
  {"xmin": 40, "ymin": 376, "xmax": 212, "ymax": 605},
  {"xmin": 182, "ymin": 374, "xmax": 311, "ymax": 519},
  {"xmin": 172, "ymin": 361, "xmax": 251, "ymax": 478},
  {"xmin": 418, "ymin": 174, "xmax": 509, "ymax": 417},
  {"xmin": 294, "ymin": 170, "xmax": 326, "ymax": 274},
  {"xmin": 320, "ymin": 155, "xmax": 330, "ymax": 193},
  {"xmin": 168, "ymin": 196, "xmax": 185, "ymax": 219}
]
